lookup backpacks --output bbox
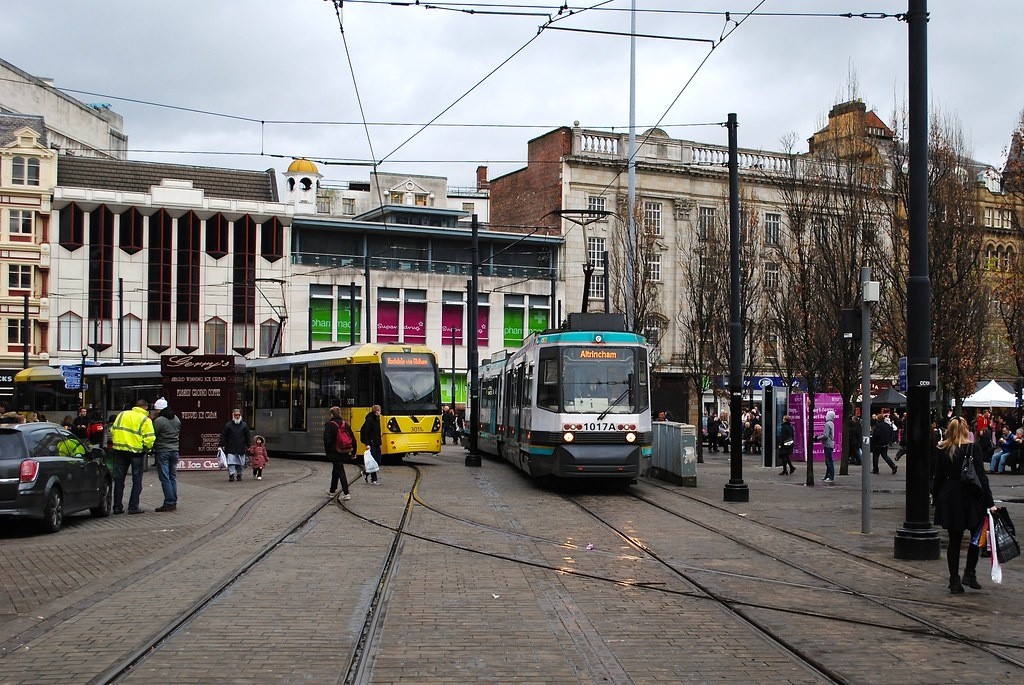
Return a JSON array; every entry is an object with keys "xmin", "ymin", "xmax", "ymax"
[{"xmin": 330, "ymin": 420, "xmax": 353, "ymax": 454}]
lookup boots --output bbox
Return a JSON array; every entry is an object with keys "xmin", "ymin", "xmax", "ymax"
[
  {"xmin": 948, "ymin": 574, "xmax": 965, "ymax": 592},
  {"xmin": 962, "ymin": 568, "xmax": 981, "ymax": 589}
]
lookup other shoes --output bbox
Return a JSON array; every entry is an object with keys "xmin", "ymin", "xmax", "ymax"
[
  {"xmin": 253, "ymin": 473, "xmax": 262, "ymax": 480},
  {"xmin": 236, "ymin": 475, "xmax": 242, "ymax": 481},
  {"xmin": 871, "ymin": 468, "xmax": 879, "ymax": 474},
  {"xmin": 819, "ymin": 477, "xmax": 834, "ymax": 483},
  {"xmin": 361, "ymin": 471, "xmax": 368, "ymax": 484},
  {"xmin": 339, "ymin": 492, "xmax": 350, "ymax": 500},
  {"xmin": 891, "ymin": 466, "xmax": 898, "ymax": 474},
  {"xmin": 325, "ymin": 489, "xmax": 335, "ymax": 498},
  {"xmin": 229, "ymin": 474, "xmax": 234, "ymax": 481},
  {"xmin": 370, "ymin": 480, "xmax": 382, "ymax": 485},
  {"xmin": 155, "ymin": 505, "xmax": 178, "ymax": 512},
  {"xmin": 130, "ymin": 509, "xmax": 145, "ymax": 514},
  {"xmin": 113, "ymin": 510, "xmax": 126, "ymax": 514}
]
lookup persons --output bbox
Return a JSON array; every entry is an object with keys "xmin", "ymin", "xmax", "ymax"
[
  {"xmin": 248, "ymin": 435, "xmax": 271, "ymax": 480},
  {"xmin": 219, "ymin": 409, "xmax": 251, "ymax": 482},
  {"xmin": 656, "ymin": 411, "xmax": 668, "ymax": 422},
  {"xmin": 152, "ymin": 396, "xmax": 182, "ymax": 512},
  {"xmin": 441, "ymin": 404, "xmax": 465, "ymax": 445},
  {"xmin": 360, "ymin": 404, "xmax": 382, "ymax": 485},
  {"xmin": 933, "ymin": 416, "xmax": 997, "ymax": 593},
  {"xmin": 323, "ymin": 406, "xmax": 357, "ymax": 501},
  {"xmin": 702, "ymin": 406, "xmax": 1024, "ymax": 483},
  {"xmin": 0, "ymin": 403, "xmax": 117, "ymax": 468},
  {"xmin": 108, "ymin": 398, "xmax": 157, "ymax": 514}
]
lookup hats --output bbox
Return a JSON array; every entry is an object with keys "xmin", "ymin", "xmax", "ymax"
[
  {"xmin": 877, "ymin": 414, "xmax": 883, "ymax": 421},
  {"xmin": 232, "ymin": 408, "xmax": 241, "ymax": 415},
  {"xmin": 154, "ymin": 397, "xmax": 167, "ymax": 409}
]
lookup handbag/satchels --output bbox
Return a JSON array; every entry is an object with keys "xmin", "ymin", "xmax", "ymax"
[
  {"xmin": 217, "ymin": 448, "xmax": 228, "ymax": 469},
  {"xmin": 960, "ymin": 443, "xmax": 983, "ymax": 492},
  {"xmin": 363, "ymin": 449, "xmax": 380, "ymax": 473},
  {"xmin": 972, "ymin": 507, "xmax": 1020, "ymax": 584},
  {"xmin": 783, "ymin": 437, "xmax": 795, "ymax": 449}
]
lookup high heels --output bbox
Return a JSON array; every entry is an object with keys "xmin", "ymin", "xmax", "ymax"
[
  {"xmin": 779, "ymin": 470, "xmax": 789, "ymax": 475},
  {"xmin": 790, "ymin": 467, "xmax": 796, "ymax": 474}
]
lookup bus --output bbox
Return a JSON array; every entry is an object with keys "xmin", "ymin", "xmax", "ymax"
[
  {"xmin": 461, "ymin": 313, "xmax": 653, "ymax": 492},
  {"xmin": 13, "ymin": 340, "xmax": 442, "ymax": 465}
]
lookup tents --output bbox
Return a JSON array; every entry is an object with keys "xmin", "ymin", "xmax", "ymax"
[
  {"xmin": 873, "ymin": 384, "xmax": 907, "ymax": 409},
  {"xmin": 951, "ymin": 378, "xmax": 1017, "ymax": 408}
]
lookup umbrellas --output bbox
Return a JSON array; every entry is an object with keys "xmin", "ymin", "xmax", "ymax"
[{"xmin": 14, "ymin": 365, "xmax": 64, "ymax": 411}]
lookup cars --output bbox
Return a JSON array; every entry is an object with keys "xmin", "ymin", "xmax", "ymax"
[
  {"xmin": 701, "ymin": 416, "xmax": 728, "ymax": 446},
  {"xmin": 0, "ymin": 422, "xmax": 113, "ymax": 531}
]
